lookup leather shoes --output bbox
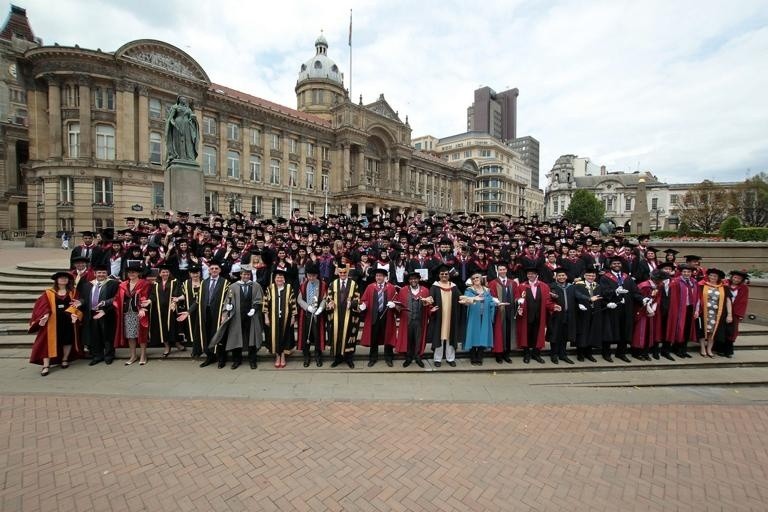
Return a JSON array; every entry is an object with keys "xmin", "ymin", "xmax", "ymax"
[
  {"xmin": 551, "ymin": 353, "xmax": 652, "ymax": 364},
  {"xmin": 41, "ymin": 367, "xmax": 49, "ymax": 376},
  {"xmin": 274, "ymin": 356, "xmax": 354, "ymax": 368},
  {"xmin": 61, "ymin": 360, "xmax": 69, "ymax": 369},
  {"xmin": 89, "ymin": 360, "xmax": 102, "ymax": 365},
  {"xmin": 652, "ymin": 348, "xmax": 734, "ymax": 361},
  {"xmin": 106, "ymin": 359, "xmax": 112, "ymax": 364},
  {"xmin": 191, "ymin": 350, "xmax": 257, "ymax": 370},
  {"xmin": 125, "ymin": 355, "xmax": 147, "ymax": 365},
  {"xmin": 469, "ymin": 353, "xmax": 545, "ymax": 366},
  {"xmin": 163, "ymin": 345, "xmax": 171, "ymax": 358},
  {"xmin": 175, "ymin": 343, "xmax": 186, "ymax": 351},
  {"xmin": 367, "ymin": 358, "xmax": 457, "ymax": 368}
]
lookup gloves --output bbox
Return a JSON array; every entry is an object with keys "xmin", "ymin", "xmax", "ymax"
[
  {"xmin": 617, "ymin": 288, "xmax": 629, "ymax": 294},
  {"xmin": 226, "ymin": 304, "xmax": 233, "ymax": 311},
  {"xmin": 607, "ymin": 302, "xmax": 617, "ymax": 309},
  {"xmin": 386, "ymin": 301, "xmax": 396, "ymax": 309},
  {"xmin": 308, "ymin": 305, "xmax": 322, "ymax": 316},
  {"xmin": 643, "ymin": 297, "xmax": 658, "ymax": 314},
  {"xmin": 578, "ymin": 304, "xmax": 587, "ymax": 311},
  {"xmin": 358, "ymin": 302, "xmax": 366, "ymax": 311},
  {"xmin": 518, "ymin": 298, "xmax": 525, "ymax": 304},
  {"xmin": 247, "ymin": 309, "xmax": 256, "ymax": 317}
]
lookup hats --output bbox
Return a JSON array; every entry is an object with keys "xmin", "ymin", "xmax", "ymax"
[
  {"xmin": 51, "ymin": 271, "xmax": 73, "ymax": 281},
  {"xmin": 74, "ymin": 228, "xmax": 127, "ymax": 271},
  {"xmin": 603, "ymin": 225, "xmax": 650, "ymax": 262},
  {"xmin": 651, "ymin": 246, "xmax": 749, "ymax": 285},
  {"xmin": 127, "ymin": 209, "xmax": 602, "ymax": 280}
]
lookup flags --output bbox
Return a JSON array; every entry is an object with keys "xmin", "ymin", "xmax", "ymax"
[{"xmin": 348, "ymin": 15, "xmax": 352, "ymax": 46}]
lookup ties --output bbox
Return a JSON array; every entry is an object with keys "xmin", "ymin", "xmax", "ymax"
[
  {"xmin": 618, "ymin": 274, "xmax": 622, "ymax": 286},
  {"xmin": 209, "ymin": 280, "xmax": 216, "ymax": 304},
  {"xmin": 308, "ymin": 283, "xmax": 316, "ymax": 305},
  {"xmin": 74, "ymin": 271, "xmax": 81, "ymax": 289},
  {"xmin": 92, "ymin": 283, "xmax": 100, "ymax": 311},
  {"xmin": 378, "ymin": 284, "xmax": 383, "ymax": 312},
  {"xmin": 340, "ymin": 282, "xmax": 346, "ymax": 303}
]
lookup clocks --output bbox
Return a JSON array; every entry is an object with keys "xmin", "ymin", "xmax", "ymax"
[{"xmin": 8, "ymin": 63, "xmax": 17, "ymax": 79}]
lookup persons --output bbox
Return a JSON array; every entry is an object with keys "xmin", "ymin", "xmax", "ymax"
[{"xmin": 162, "ymin": 95, "xmax": 200, "ymax": 162}]
